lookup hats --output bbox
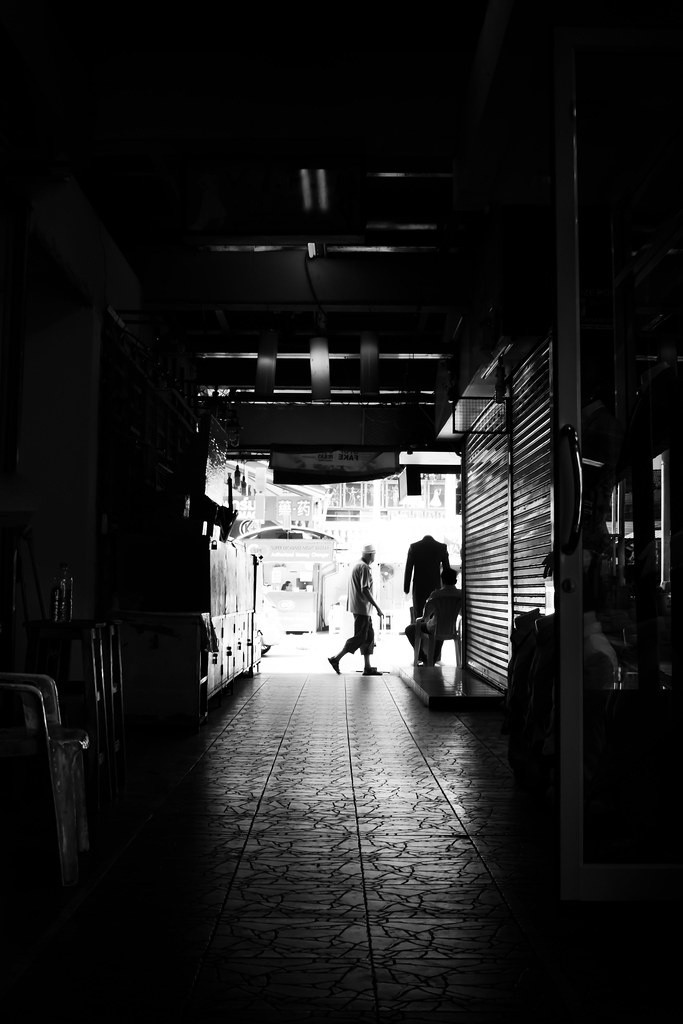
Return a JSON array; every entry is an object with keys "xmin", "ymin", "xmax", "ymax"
[{"xmin": 362, "ymin": 543, "xmax": 376, "ymax": 553}]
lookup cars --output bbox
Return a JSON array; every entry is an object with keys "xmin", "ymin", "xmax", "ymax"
[{"xmin": 233, "ymin": 524, "xmax": 347, "ymax": 591}]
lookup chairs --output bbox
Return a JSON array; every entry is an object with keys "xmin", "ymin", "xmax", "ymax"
[
  {"xmin": 413, "ymin": 590, "xmax": 463, "ymax": 670},
  {"xmin": 0, "ymin": 672, "xmax": 91, "ymax": 888}
]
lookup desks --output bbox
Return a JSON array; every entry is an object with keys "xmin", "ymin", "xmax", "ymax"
[{"xmin": 21, "ymin": 618, "xmax": 129, "ymax": 813}]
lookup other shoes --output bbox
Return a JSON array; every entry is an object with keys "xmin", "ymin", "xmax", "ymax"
[
  {"xmin": 327, "ymin": 656, "xmax": 341, "ymax": 675},
  {"xmin": 362, "ymin": 667, "xmax": 382, "ymax": 675}
]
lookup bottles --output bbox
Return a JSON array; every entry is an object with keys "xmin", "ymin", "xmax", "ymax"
[{"xmin": 50, "ymin": 561, "xmax": 73, "ymax": 624}]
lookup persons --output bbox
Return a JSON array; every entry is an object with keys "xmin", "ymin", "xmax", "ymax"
[
  {"xmin": 326, "ymin": 544, "xmax": 384, "ymax": 676},
  {"xmin": 502, "ymin": 610, "xmax": 620, "ymax": 819},
  {"xmin": 405, "ymin": 568, "xmax": 462, "ymax": 666},
  {"xmin": 404, "ymin": 535, "xmax": 451, "ymax": 624}
]
